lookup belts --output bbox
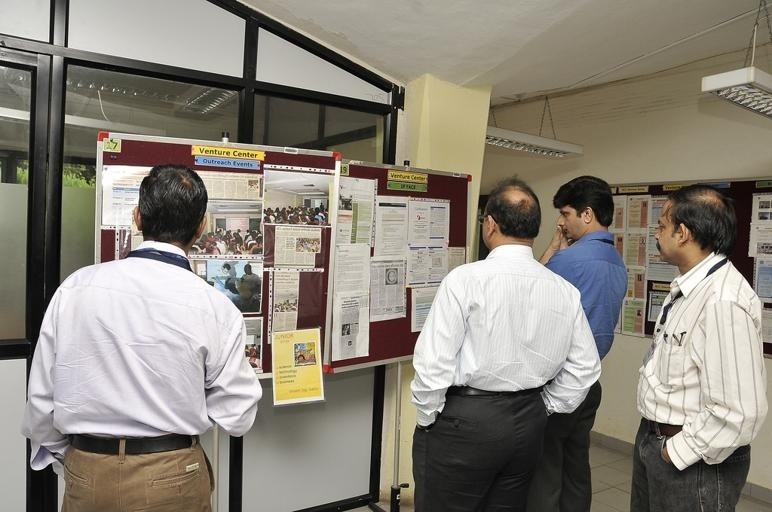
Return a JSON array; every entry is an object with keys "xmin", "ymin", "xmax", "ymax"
[
  {"xmin": 68, "ymin": 432, "xmax": 192, "ymax": 457},
  {"xmin": 646, "ymin": 420, "xmax": 684, "ymax": 437},
  {"xmin": 446, "ymin": 384, "xmax": 500, "ymax": 397}
]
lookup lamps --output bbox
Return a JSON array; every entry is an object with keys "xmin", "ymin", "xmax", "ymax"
[
  {"xmin": 486, "ymin": 96, "xmax": 583, "ymax": 161},
  {"xmin": 702, "ymin": 0, "xmax": 772, "ymax": 118}
]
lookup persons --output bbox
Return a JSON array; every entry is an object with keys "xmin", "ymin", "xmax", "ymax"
[
  {"xmin": 264, "ymin": 200, "xmax": 327, "ymax": 224},
  {"xmin": 225, "ymin": 263, "xmax": 260, "ymax": 311},
  {"xmin": 409, "ymin": 177, "xmax": 602, "ymax": 512},
  {"xmin": 20, "ymin": 161, "xmax": 262, "ymax": 512},
  {"xmin": 631, "ymin": 183, "xmax": 769, "ymax": 512},
  {"xmin": 526, "ymin": 175, "xmax": 628, "ymax": 512},
  {"xmin": 190, "ymin": 228, "xmax": 263, "ymax": 255},
  {"xmin": 245, "ymin": 343, "xmax": 261, "ymax": 367}
]
